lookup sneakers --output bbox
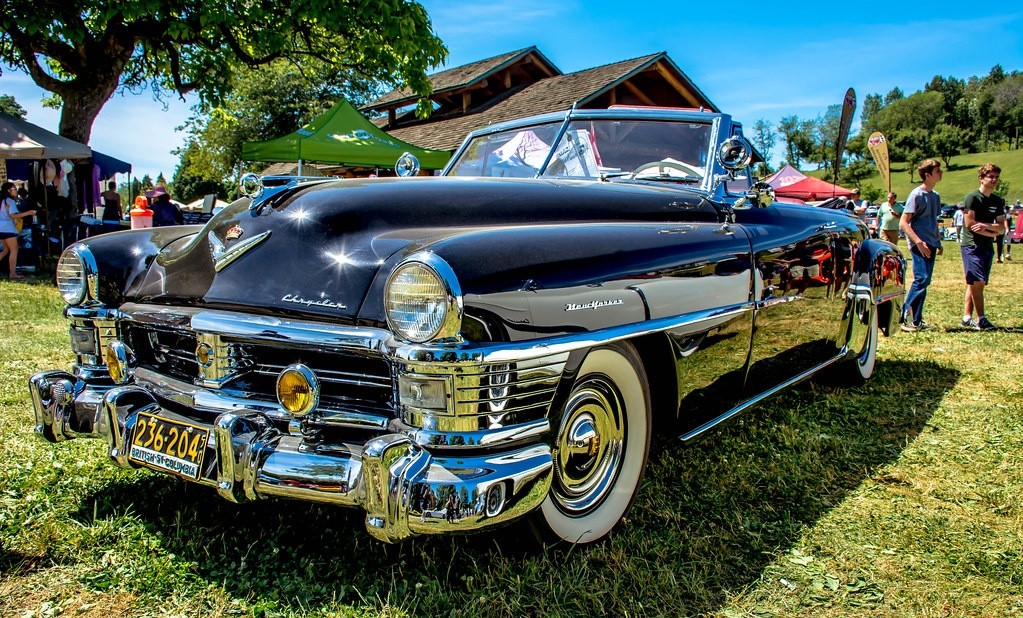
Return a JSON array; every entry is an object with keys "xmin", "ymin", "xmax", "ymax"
[
  {"xmin": 917, "ymin": 321, "xmax": 936, "ymax": 331},
  {"xmin": 978, "ymin": 318, "xmax": 998, "ymax": 331},
  {"xmin": 961, "ymin": 318, "xmax": 980, "ymax": 331},
  {"xmin": 900, "ymin": 321, "xmax": 918, "ymax": 332}
]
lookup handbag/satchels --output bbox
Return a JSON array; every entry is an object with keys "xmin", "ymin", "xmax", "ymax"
[{"xmin": 14, "ymin": 217, "xmax": 23, "ymax": 232}]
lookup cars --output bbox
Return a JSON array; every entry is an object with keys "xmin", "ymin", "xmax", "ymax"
[
  {"xmin": 939, "ymin": 203, "xmax": 958, "ymax": 218},
  {"xmin": 898, "ymin": 201, "xmax": 907, "ymax": 208},
  {"xmin": 30, "ymin": 102, "xmax": 907, "ymax": 551},
  {"xmin": 865, "ymin": 205, "xmax": 880, "ymax": 215}
]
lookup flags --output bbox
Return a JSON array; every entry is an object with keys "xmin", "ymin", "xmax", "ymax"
[
  {"xmin": 868, "ymin": 131, "xmax": 890, "ymax": 192},
  {"xmin": 835, "ymin": 87, "xmax": 856, "ymax": 172}
]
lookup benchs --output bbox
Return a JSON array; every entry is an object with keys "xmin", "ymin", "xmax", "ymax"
[{"xmin": 180, "ymin": 212, "xmax": 213, "ymax": 224}]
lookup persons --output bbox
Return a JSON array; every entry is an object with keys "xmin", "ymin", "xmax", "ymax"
[
  {"xmin": 146, "ymin": 185, "xmax": 183, "ymax": 226},
  {"xmin": 961, "ymin": 164, "xmax": 1005, "ymax": 329},
  {"xmin": 952, "ymin": 202, "xmax": 963, "ymax": 242},
  {"xmin": 761, "ymin": 234, "xmax": 903, "ymax": 299},
  {"xmin": 98, "ymin": 182, "xmax": 124, "ymax": 221},
  {"xmin": 996, "ymin": 206, "xmax": 1012, "ymax": 263},
  {"xmin": 899, "ymin": 160, "xmax": 944, "ymax": 331},
  {"xmin": 0, "ymin": 182, "xmax": 37, "ymax": 278},
  {"xmin": 839, "ymin": 188, "xmax": 867, "ymax": 223},
  {"xmin": 875, "ymin": 193, "xmax": 903, "ymax": 244},
  {"xmin": 807, "ymin": 192, "xmax": 816, "ymax": 201}
]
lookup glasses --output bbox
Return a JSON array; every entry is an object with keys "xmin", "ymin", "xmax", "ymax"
[{"xmin": 10, "ymin": 188, "xmax": 16, "ymax": 190}]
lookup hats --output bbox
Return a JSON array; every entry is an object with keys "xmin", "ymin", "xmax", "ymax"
[
  {"xmin": 807, "ymin": 192, "xmax": 816, "ymax": 198},
  {"xmin": 1005, "ymin": 205, "xmax": 1011, "ymax": 210},
  {"xmin": 957, "ymin": 201, "xmax": 965, "ymax": 209},
  {"xmin": 146, "ymin": 184, "xmax": 168, "ymax": 197},
  {"xmin": 850, "ymin": 188, "xmax": 860, "ymax": 195}
]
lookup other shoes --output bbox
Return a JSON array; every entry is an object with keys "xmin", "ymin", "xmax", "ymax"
[
  {"xmin": 1005, "ymin": 254, "xmax": 1012, "ymax": 261},
  {"xmin": 997, "ymin": 259, "xmax": 1003, "ymax": 263},
  {"xmin": 1001, "ymin": 254, "xmax": 1004, "ymax": 261},
  {"xmin": 9, "ymin": 274, "xmax": 24, "ymax": 280}
]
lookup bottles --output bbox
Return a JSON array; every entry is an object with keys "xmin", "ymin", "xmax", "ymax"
[
  {"xmin": 130, "ymin": 196, "xmax": 154, "ymax": 230},
  {"xmin": 33, "ymin": 210, "xmax": 38, "ymax": 224}
]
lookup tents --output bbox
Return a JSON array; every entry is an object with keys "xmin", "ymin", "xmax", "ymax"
[
  {"xmin": 459, "ymin": 130, "xmax": 622, "ymax": 177},
  {"xmin": 0, "ymin": 111, "xmax": 93, "ymax": 269},
  {"xmin": 766, "ymin": 165, "xmax": 852, "ymax": 199},
  {"xmin": 238, "ymin": 99, "xmax": 451, "ymax": 198},
  {"xmin": 90, "ymin": 148, "xmax": 132, "ymax": 205}
]
